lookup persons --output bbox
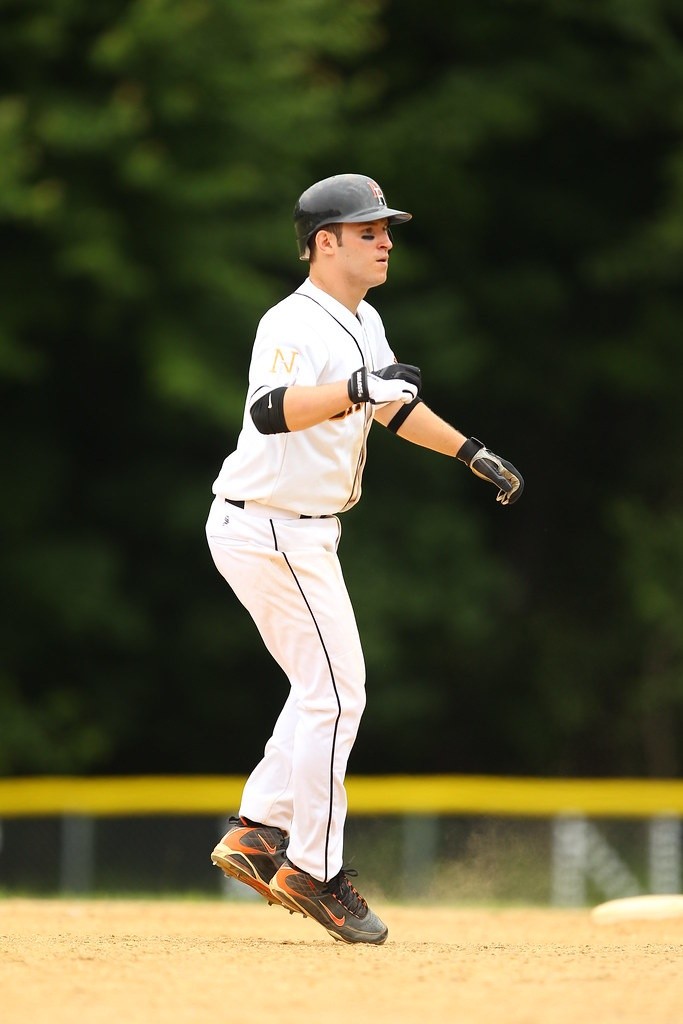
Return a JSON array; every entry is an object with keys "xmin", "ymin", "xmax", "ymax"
[{"xmin": 205, "ymin": 173, "xmax": 524, "ymax": 945}]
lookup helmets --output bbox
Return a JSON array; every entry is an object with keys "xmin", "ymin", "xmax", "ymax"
[{"xmin": 292, "ymin": 174, "xmax": 412, "ymax": 261}]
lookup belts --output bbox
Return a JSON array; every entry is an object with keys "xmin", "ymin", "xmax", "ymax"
[{"xmin": 226, "ymin": 501, "xmax": 330, "ymax": 522}]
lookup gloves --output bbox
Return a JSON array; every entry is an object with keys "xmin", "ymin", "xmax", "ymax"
[
  {"xmin": 455, "ymin": 438, "xmax": 525, "ymax": 506},
  {"xmin": 348, "ymin": 362, "xmax": 423, "ymax": 405}
]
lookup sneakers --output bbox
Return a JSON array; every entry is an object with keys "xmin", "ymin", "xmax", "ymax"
[
  {"xmin": 268, "ymin": 861, "xmax": 389, "ymax": 947},
  {"xmin": 210, "ymin": 817, "xmax": 312, "ymax": 919}
]
